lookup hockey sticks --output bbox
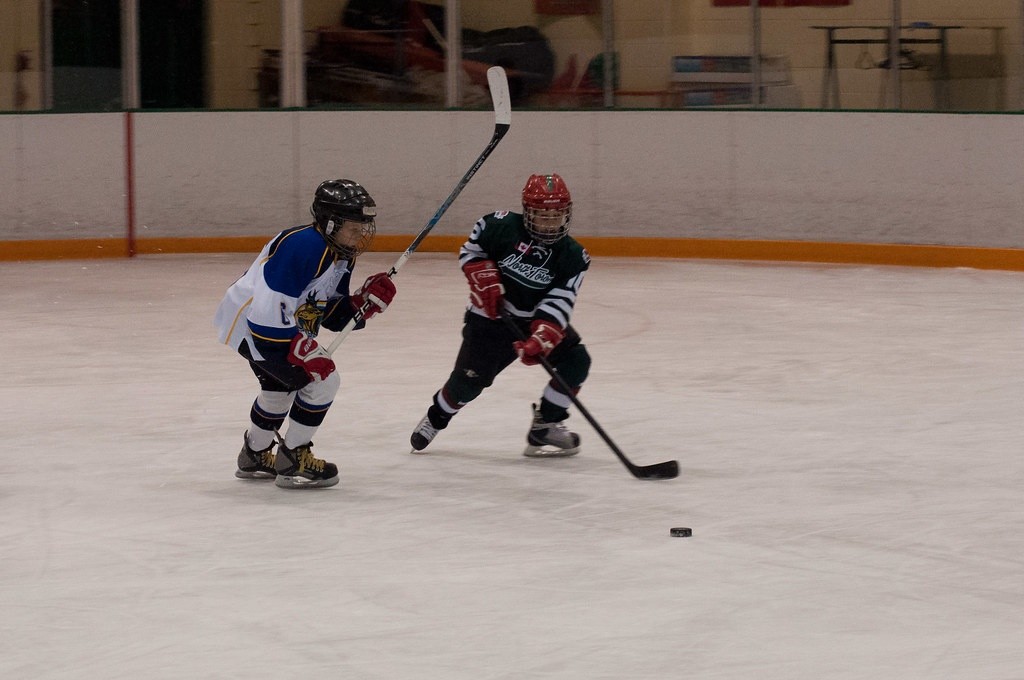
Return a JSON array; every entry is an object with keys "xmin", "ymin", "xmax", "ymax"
[
  {"xmin": 325, "ymin": 64, "xmax": 514, "ymax": 356},
  {"xmin": 495, "ymin": 302, "xmax": 681, "ymax": 483}
]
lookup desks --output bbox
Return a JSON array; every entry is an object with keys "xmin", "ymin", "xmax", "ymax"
[{"xmin": 808, "ymin": 23, "xmax": 963, "ymax": 109}]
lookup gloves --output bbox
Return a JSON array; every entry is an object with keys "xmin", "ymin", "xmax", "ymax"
[
  {"xmin": 513, "ymin": 319, "xmax": 567, "ymax": 366},
  {"xmin": 350, "ymin": 272, "xmax": 396, "ymax": 321},
  {"xmin": 287, "ymin": 331, "xmax": 336, "ymax": 383},
  {"xmin": 461, "ymin": 260, "xmax": 506, "ymax": 321}
]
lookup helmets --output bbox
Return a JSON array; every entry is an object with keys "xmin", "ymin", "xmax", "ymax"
[
  {"xmin": 522, "ymin": 171, "xmax": 573, "ymax": 245},
  {"xmin": 309, "ymin": 178, "xmax": 377, "ymax": 259}
]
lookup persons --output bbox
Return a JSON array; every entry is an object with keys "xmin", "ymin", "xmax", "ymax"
[
  {"xmin": 410, "ymin": 173, "xmax": 592, "ymax": 458},
  {"xmin": 211, "ymin": 178, "xmax": 397, "ymax": 489}
]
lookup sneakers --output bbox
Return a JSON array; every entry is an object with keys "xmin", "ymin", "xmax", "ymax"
[
  {"xmin": 273, "ymin": 426, "xmax": 339, "ymax": 489},
  {"xmin": 410, "ymin": 406, "xmax": 451, "ymax": 453},
  {"xmin": 523, "ymin": 403, "xmax": 580, "ymax": 457},
  {"xmin": 235, "ymin": 430, "xmax": 277, "ymax": 479}
]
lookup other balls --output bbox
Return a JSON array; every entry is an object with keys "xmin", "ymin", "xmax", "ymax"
[{"xmin": 669, "ymin": 527, "xmax": 693, "ymax": 538}]
